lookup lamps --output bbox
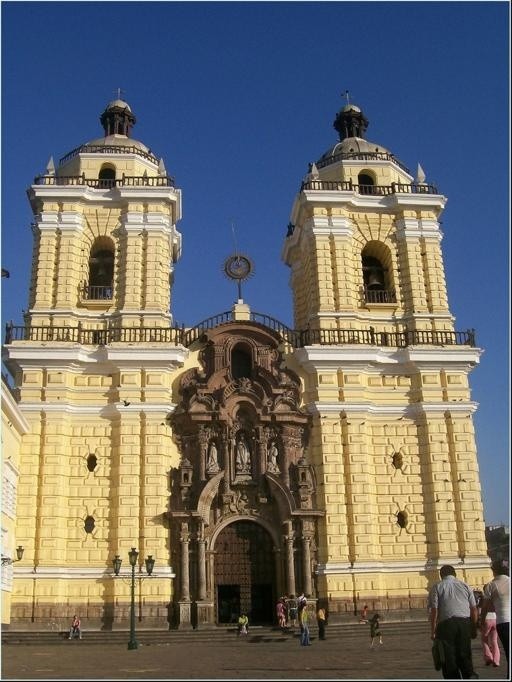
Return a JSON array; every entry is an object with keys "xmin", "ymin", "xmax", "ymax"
[{"xmin": 1, "ymin": 545, "xmax": 25, "ymax": 568}]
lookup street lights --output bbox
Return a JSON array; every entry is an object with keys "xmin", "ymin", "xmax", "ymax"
[{"xmin": 112, "ymin": 548, "xmax": 155, "ymax": 650}]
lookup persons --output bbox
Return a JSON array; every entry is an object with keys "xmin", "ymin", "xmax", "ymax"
[
  {"xmin": 479, "ymin": 559, "xmax": 509, "ymax": 678},
  {"xmin": 278, "ymin": 591, "xmax": 307, "ymax": 627},
  {"xmin": 238, "ymin": 612, "xmax": 250, "ymax": 635},
  {"xmin": 68, "ymin": 614, "xmax": 82, "ymax": 639},
  {"xmin": 428, "ymin": 564, "xmax": 479, "ymax": 679},
  {"xmin": 359, "ymin": 605, "xmax": 368, "ymax": 623},
  {"xmin": 363, "ymin": 612, "xmax": 384, "ymax": 647},
  {"xmin": 207, "ymin": 442, "xmax": 221, "ymax": 470},
  {"xmin": 299, "ymin": 603, "xmax": 313, "ymax": 646},
  {"xmin": 267, "ymin": 441, "xmax": 280, "ymax": 473},
  {"xmin": 318, "ymin": 605, "xmax": 328, "ymax": 639},
  {"xmin": 476, "ymin": 582, "xmax": 500, "ymax": 667},
  {"xmin": 236, "ymin": 432, "xmax": 252, "ymax": 469}
]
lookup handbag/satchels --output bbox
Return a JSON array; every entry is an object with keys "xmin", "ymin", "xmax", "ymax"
[{"xmin": 432, "ymin": 639, "xmax": 445, "ymax": 671}]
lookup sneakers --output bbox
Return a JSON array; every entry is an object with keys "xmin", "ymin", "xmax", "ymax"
[
  {"xmin": 300, "ymin": 643, "xmax": 312, "ymax": 646},
  {"xmin": 485, "ymin": 659, "xmax": 499, "ymax": 667}
]
